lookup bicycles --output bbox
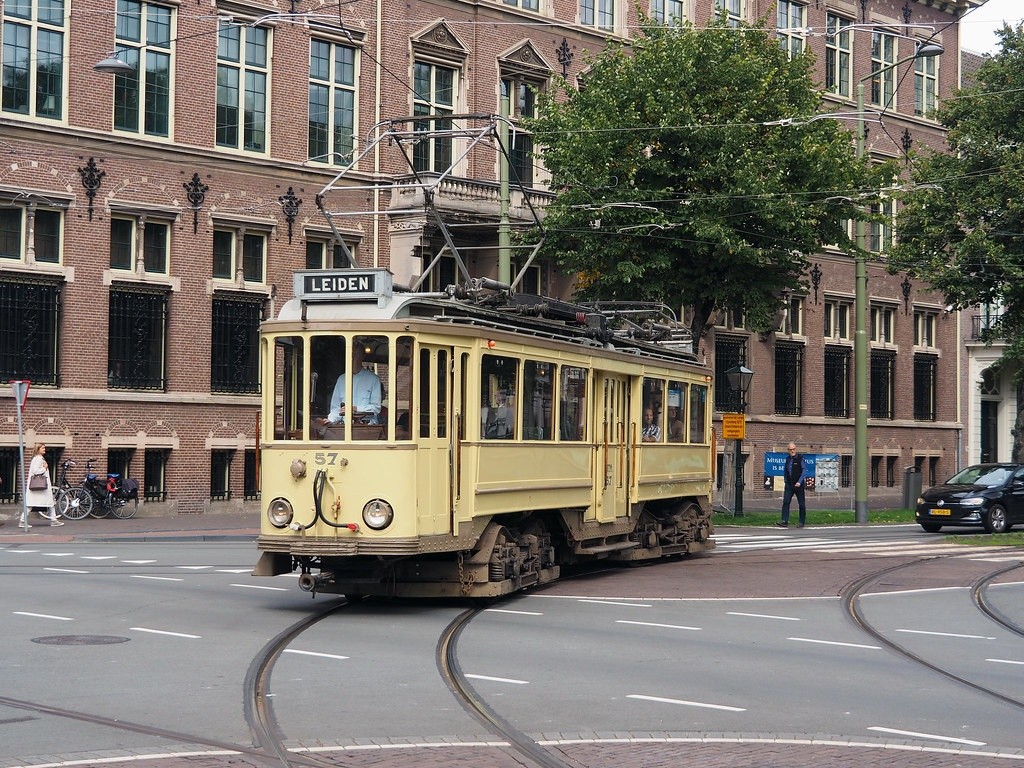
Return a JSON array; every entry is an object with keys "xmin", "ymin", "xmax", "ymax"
[{"xmin": 39, "ymin": 459, "xmax": 139, "ymax": 521}]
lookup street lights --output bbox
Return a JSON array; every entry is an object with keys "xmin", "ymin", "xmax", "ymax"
[
  {"xmin": 854, "ymin": 46, "xmax": 946, "ymax": 524},
  {"xmin": 725, "ymin": 362, "xmax": 755, "ymax": 518}
]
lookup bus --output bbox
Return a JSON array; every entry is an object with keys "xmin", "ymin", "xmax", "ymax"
[{"xmin": 253, "ymin": 267, "xmax": 720, "ymax": 601}]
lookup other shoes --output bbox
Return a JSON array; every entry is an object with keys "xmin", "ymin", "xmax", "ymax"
[
  {"xmin": 776, "ymin": 521, "xmax": 788, "ymax": 527},
  {"xmin": 795, "ymin": 523, "xmax": 804, "ymax": 527}
]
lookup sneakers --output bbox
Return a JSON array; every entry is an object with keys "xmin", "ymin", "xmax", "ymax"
[
  {"xmin": 19, "ymin": 522, "xmax": 32, "ymax": 527},
  {"xmin": 51, "ymin": 521, "xmax": 64, "ymax": 526}
]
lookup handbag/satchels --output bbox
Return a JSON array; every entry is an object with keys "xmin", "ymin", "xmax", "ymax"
[
  {"xmin": 29, "ymin": 473, "xmax": 48, "ymax": 490},
  {"xmin": 86, "ymin": 476, "xmax": 140, "ymax": 501}
]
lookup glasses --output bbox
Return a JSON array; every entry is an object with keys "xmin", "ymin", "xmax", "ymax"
[{"xmin": 789, "ymin": 448, "xmax": 796, "ymax": 450}]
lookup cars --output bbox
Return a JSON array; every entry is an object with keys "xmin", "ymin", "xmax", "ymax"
[{"xmin": 915, "ymin": 462, "xmax": 1024, "ymax": 534}]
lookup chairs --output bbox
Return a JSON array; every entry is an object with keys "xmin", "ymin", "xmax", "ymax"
[{"xmin": 396, "ymin": 408, "xmax": 547, "ymax": 442}]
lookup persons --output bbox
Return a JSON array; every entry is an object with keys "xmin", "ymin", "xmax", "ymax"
[
  {"xmin": 776, "ymin": 443, "xmax": 806, "ymax": 528},
  {"xmin": 323, "ymin": 344, "xmax": 381, "ymax": 425},
  {"xmin": 19, "ymin": 443, "xmax": 64, "ymax": 528},
  {"xmin": 642, "ymin": 408, "xmax": 661, "ymax": 441},
  {"xmin": 668, "ymin": 406, "xmax": 684, "ymax": 441},
  {"xmin": 481, "ymin": 388, "xmax": 515, "ymax": 435}
]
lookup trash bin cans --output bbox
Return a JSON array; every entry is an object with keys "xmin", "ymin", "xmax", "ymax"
[{"xmin": 902, "ymin": 465, "xmax": 923, "ymax": 510}]
